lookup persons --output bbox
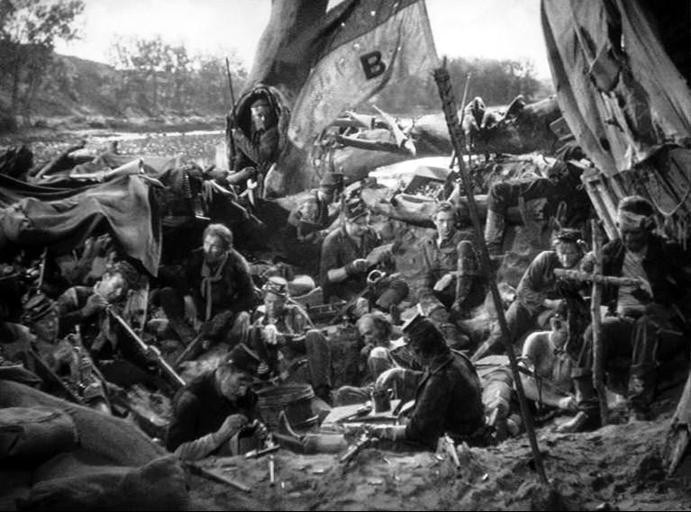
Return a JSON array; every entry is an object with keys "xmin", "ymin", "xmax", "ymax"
[{"xmin": 221, "ymin": 97, "xmax": 280, "ymax": 188}]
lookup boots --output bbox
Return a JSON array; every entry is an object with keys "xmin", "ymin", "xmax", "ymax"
[
  {"xmin": 450, "ymin": 278, "xmax": 473, "ymax": 321},
  {"xmin": 629, "ymin": 362, "xmax": 659, "ymax": 422},
  {"xmin": 278, "ymin": 411, "xmax": 300, "ymax": 441},
  {"xmin": 557, "ymin": 384, "xmax": 599, "ymax": 432}
]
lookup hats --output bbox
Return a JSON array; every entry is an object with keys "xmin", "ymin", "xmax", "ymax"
[
  {"xmin": 345, "ymin": 198, "xmax": 368, "ymax": 223},
  {"xmin": 262, "ymin": 277, "xmax": 288, "ymax": 296},
  {"xmin": 21, "ymin": 295, "xmax": 55, "ymax": 323},
  {"xmin": 557, "ymin": 228, "xmax": 581, "ymax": 242},
  {"xmin": 225, "ymin": 343, "xmax": 261, "ymax": 375}
]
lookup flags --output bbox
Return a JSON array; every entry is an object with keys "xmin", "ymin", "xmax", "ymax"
[{"xmin": 268, "ymin": 1, "xmax": 439, "ymax": 149}]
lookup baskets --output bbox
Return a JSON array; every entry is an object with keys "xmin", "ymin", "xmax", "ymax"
[{"xmin": 255, "ymin": 384, "xmax": 319, "ymax": 432}]
